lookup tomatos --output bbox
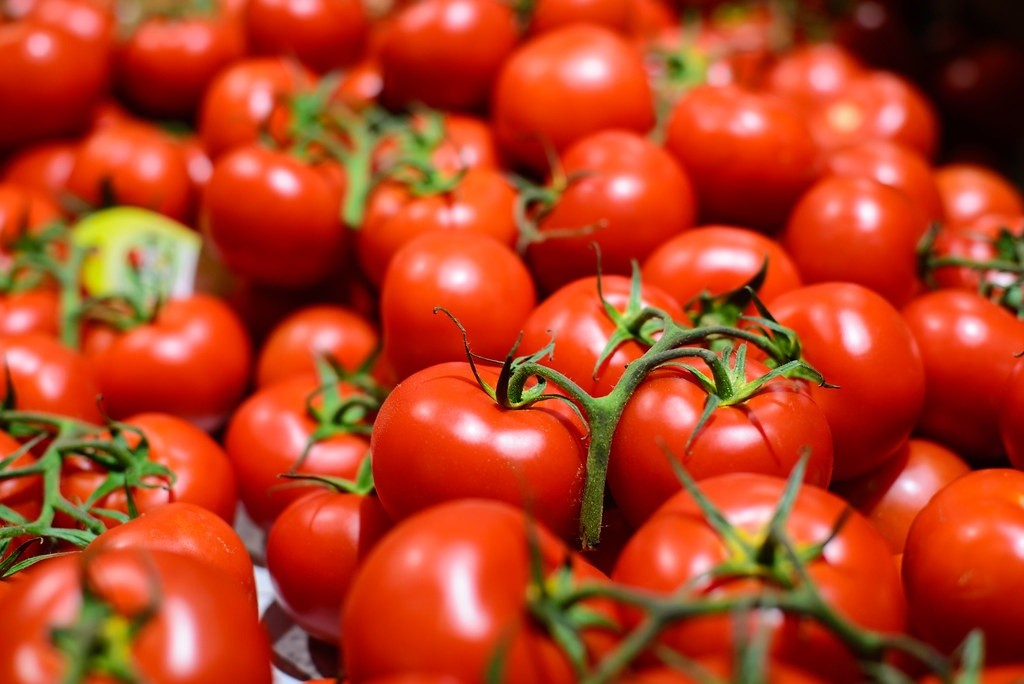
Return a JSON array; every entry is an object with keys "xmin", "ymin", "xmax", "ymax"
[{"xmin": 0, "ymin": 0, "xmax": 1024, "ymax": 684}]
